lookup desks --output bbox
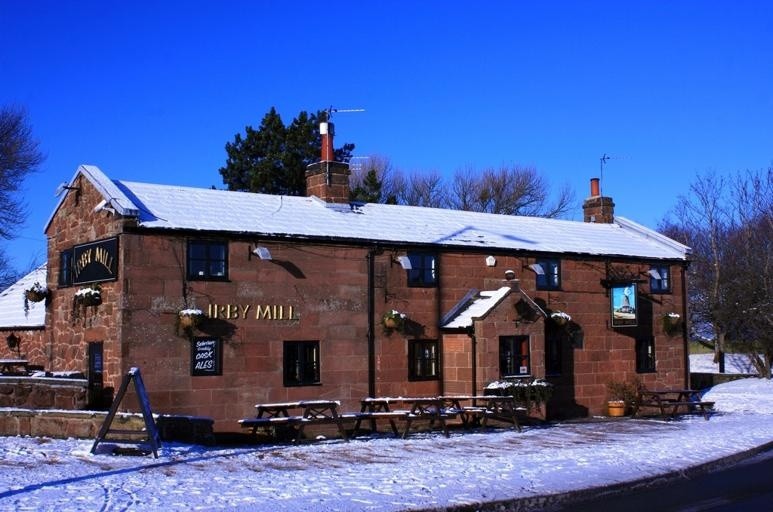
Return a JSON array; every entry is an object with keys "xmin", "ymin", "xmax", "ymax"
[{"xmin": 640, "ymin": 389, "xmax": 709, "ymax": 421}]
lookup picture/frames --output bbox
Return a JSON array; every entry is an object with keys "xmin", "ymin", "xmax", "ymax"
[
  {"xmin": 191, "ymin": 336, "xmax": 222, "ymax": 376},
  {"xmin": 610, "ymin": 283, "xmax": 638, "ymax": 328}
]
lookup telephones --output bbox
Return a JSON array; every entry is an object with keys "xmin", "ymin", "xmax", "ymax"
[{"xmin": 133, "ymin": 367, "xmax": 159, "ymax": 442}]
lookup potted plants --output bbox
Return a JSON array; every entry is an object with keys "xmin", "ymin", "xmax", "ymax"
[
  {"xmin": 663, "ymin": 312, "xmax": 682, "ymax": 336},
  {"xmin": 606, "ymin": 376, "xmax": 649, "ymax": 416},
  {"xmin": 381, "ymin": 310, "xmax": 408, "ymax": 335},
  {"xmin": 175, "ymin": 308, "xmax": 203, "ymax": 327},
  {"xmin": 24, "ymin": 282, "xmax": 48, "ymax": 316},
  {"xmin": 71, "ymin": 282, "xmax": 102, "ymax": 326}
]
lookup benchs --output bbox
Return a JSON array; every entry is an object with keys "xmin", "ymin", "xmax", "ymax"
[
  {"xmin": 667, "ymin": 402, "xmax": 715, "ymax": 421},
  {"xmin": 239, "ymin": 394, "xmax": 528, "ymax": 445}
]
